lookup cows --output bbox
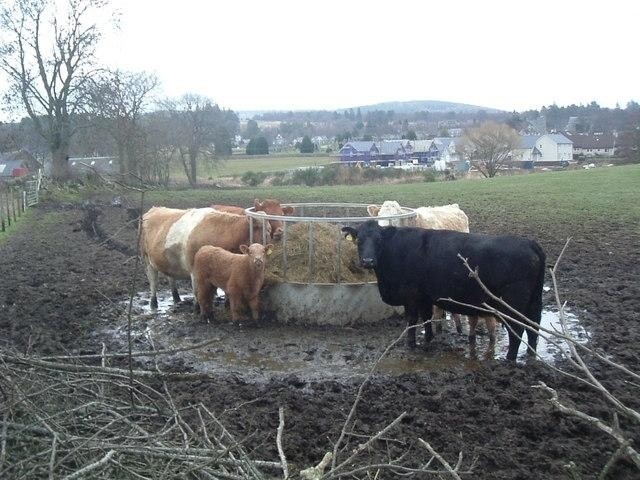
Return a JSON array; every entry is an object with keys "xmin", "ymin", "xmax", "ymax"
[
  {"xmin": 193, "ymin": 243, "xmax": 274, "ymax": 326},
  {"xmin": 340, "ymin": 220, "xmax": 547, "ymax": 361},
  {"xmin": 137, "ymin": 206, "xmax": 272, "ymax": 311},
  {"xmin": 210, "ymin": 198, "xmax": 294, "ymax": 241},
  {"xmin": 366, "ymin": 200, "xmax": 497, "ymax": 343}
]
